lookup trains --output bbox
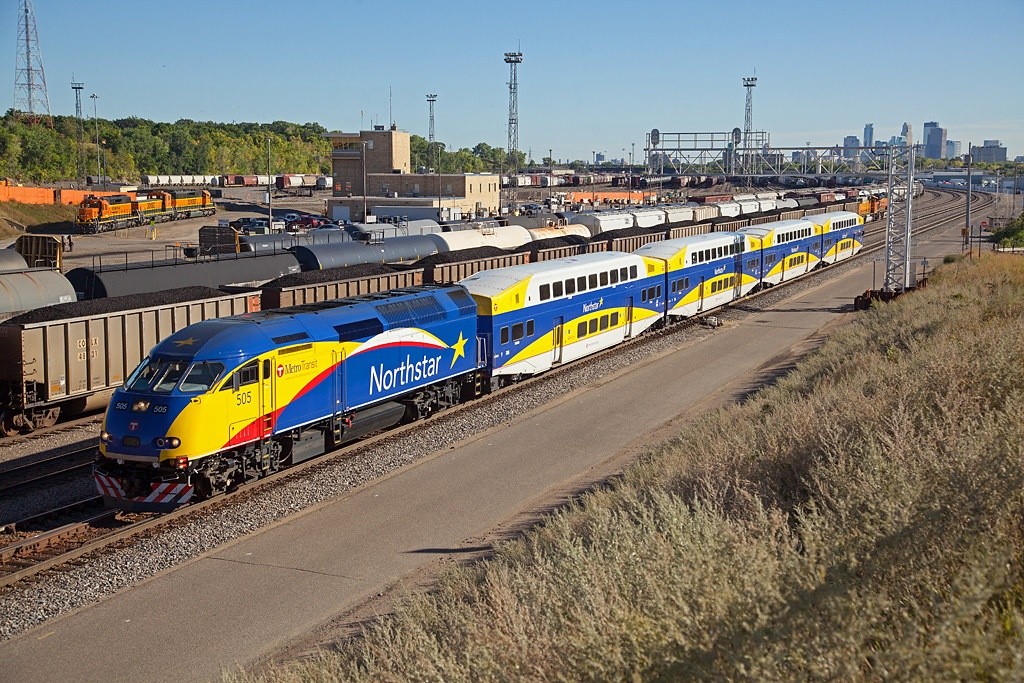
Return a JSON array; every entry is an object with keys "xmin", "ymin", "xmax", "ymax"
[
  {"xmin": 90, "ymin": 209, "xmax": 865, "ymax": 507},
  {"xmin": 752, "ymin": 176, "xmax": 865, "ymax": 188},
  {"xmin": 73, "ymin": 190, "xmax": 215, "ymax": 234},
  {"xmin": 506, "ymin": 173, "xmax": 726, "ymax": 188},
  {"xmin": 0, "ymin": 185, "xmax": 905, "ymax": 320},
  {"xmin": 0, "ymin": 195, "xmax": 887, "ymax": 438},
  {"xmin": 87, "ymin": 175, "xmax": 333, "ymax": 189}
]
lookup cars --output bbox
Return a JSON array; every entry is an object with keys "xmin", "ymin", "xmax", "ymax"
[
  {"xmin": 272, "ymin": 214, "xmax": 370, "ymax": 239},
  {"xmin": 517, "ymin": 204, "xmax": 541, "ymax": 215}
]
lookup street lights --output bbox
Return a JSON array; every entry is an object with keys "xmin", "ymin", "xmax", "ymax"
[
  {"xmin": 359, "ymin": 140, "xmax": 367, "ymax": 224},
  {"xmin": 593, "ymin": 151, "xmax": 596, "ymax": 211},
  {"xmin": 438, "ymin": 144, "xmax": 443, "ymax": 221},
  {"xmin": 549, "ymin": 149, "xmax": 553, "ymax": 214},
  {"xmin": 265, "ymin": 136, "xmax": 273, "ymax": 233},
  {"xmin": 629, "ymin": 152, "xmax": 632, "ymax": 204},
  {"xmin": 499, "ymin": 147, "xmax": 504, "ymax": 217}
]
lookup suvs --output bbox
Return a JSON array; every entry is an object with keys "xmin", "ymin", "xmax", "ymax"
[{"xmin": 227, "ymin": 217, "xmax": 267, "ymax": 231}]
